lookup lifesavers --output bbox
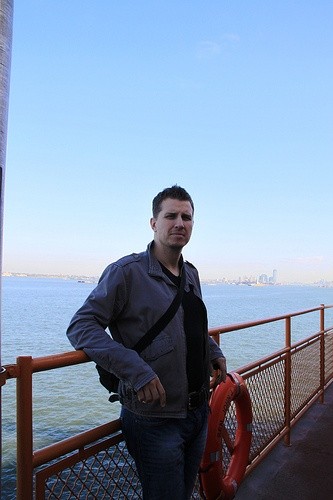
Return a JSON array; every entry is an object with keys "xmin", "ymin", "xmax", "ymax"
[{"xmin": 198, "ymin": 372, "xmax": 253, "ymax": 499}]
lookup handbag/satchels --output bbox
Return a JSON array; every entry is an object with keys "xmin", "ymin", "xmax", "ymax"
[{"xmin": 95, "ymin": 262, "xmax": 187, "ymax": 393}]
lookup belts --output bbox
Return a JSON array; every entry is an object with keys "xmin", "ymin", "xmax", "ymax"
[{"xmin": 187, "ymin": 389, "xmax": 206, "ymax": 411}]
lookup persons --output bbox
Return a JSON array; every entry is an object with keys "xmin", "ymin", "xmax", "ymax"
[{"xmin": 66, "ymin": 183, "xmax": 227, "ymax": 500}]
